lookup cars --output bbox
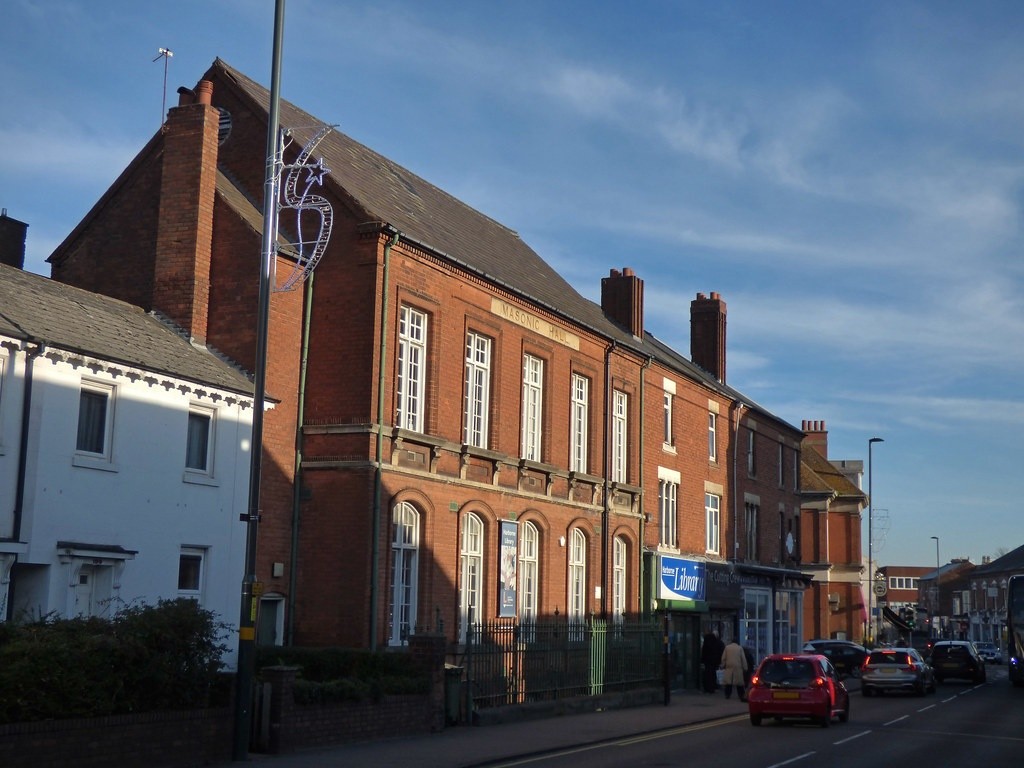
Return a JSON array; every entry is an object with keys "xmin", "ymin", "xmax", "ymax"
[
  {"xmin": 860, "ymin": 646, "xmax": 935, "ymax": 693},
  {"xmin": 804, "ymin": 642, "xmax": 872, "ymax": 677},
  {"xmin": 747, "ymin": 653, "xmax": 848, "ymax": 727},
  {"xmin": 927, "ymin": 640, "xmax": 984, "ymax": 685},
  {"xmin": 973, "ymin": 641, "xmax": 1003, "ymax": 665}
]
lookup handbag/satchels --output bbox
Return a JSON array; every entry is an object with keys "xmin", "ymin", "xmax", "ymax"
[{"xmin": 716, "ymin": 663, "xmax": 733, "ymax": 685}]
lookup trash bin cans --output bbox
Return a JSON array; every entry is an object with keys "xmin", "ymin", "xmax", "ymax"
[{"xmin": 444, "ymin": 666, "xmax": 463, "ymax": 724}]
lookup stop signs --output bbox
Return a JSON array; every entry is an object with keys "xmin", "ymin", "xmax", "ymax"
[{"xmin": 961, "ymin": 622, "xmax": 968, "ymax": 630}]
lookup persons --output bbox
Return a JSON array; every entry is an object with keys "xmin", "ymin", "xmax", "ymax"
[
  {"xmin": 896, "ymin": 637, "xmax": 907, "ymax": 647},
  {"xmin": 743, "ymin": 647, "xmax": 755, "ymax": 688},
  {"xmin": 721, "ymin": 638, "xmax": 748, "ymax": 699},
  {"xmin": 701, "ymin": 631, "xmax": 724, "ymax": 694}
]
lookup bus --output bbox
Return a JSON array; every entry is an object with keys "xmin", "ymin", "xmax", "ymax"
[{"xmin": 1001, "ymin": 575, "xmax": 1024, "ymax": 687}]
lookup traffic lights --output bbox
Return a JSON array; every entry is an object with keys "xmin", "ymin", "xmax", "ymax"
[
  {"xmin": 906, "ymin": 610, "xmax": 914, "ymax": 629},
  {"xmin": 926, "ymin": 619, "xmax": 929, "ymax": 623}
]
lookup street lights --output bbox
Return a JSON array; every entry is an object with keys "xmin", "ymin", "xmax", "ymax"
[
  {"xmin": 932, "ymin": 537, "xmax": 941, "ymax": 640},
  {"xmin": 868, "ymin": 437, "xmax": 883, "ymax": 643}
]
